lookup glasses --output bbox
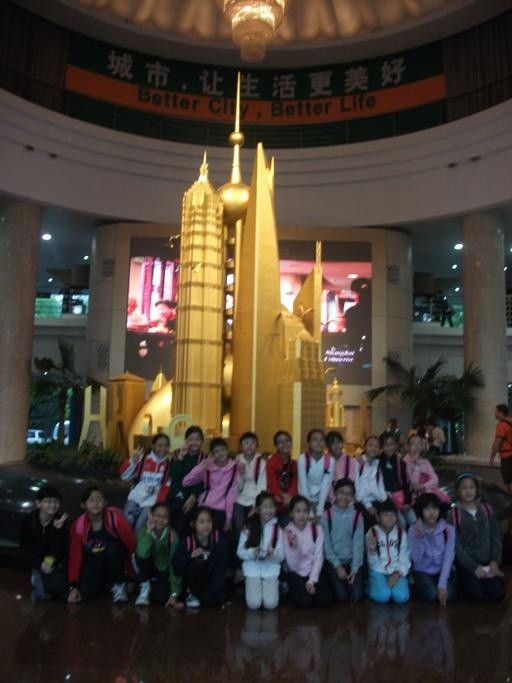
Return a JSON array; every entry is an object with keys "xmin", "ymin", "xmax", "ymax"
[{"xmin": 41, "ymin": 498, "xmax": 60, "ymax": 504}]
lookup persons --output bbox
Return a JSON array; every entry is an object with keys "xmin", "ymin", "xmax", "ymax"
[
  {"xmin": 489, "ymin": 404, "xmax": 512, "ymax": 498},
  {"xmin": 283, "ymin": 495, "xmax": 323, "ymax": 605},
  {"xmin": 400, "ymin": 432, "xmax": 454, "ymax": 526},
  {"xmin": 130, "ymin": 502, "xmax": 185, "ymax": 608},
  {"xmin": 345, "ymin": 278, "xmax": 371, "ymax": 384},
  {"xmin": 68, "ymin": 486, "xmax": 135, "ymax": 603},
  {"xmin": 128, "ymin": 297, "xmax": 147, "ymax": 332},
  {"xmin": 366, "ymin": 500, "xmax": 412, "ymax": 604},
  {"xmin": 408, "ymin": 494, "xmax": 456, "ymax": 606},
  {"xmin": 165, "ymin": 426, "xmax": 208, "ymax": 524},
  {"xmin": 428, "ymin": 422, "xmax": 446, "ymax": 455},
  {"xmin": 298, "ymin": 428, "xmax": 336, "ymax": 524},
  {"xmin": 172, "ymin": 506, "xmax": 232, "ymax": 608},
  {"xmin": 235, "ymin": 432, "xmax": 267, "ymax": 551},
  {"xmin": 236, "ymin": 491, "xmax": 285, "ymax": 610},
  {"xmin": 268, "ymin": 430, "xmax": 298, "ymax": 531},
  {"xmin": 117, "ymin": 434, "xmax": 172, "ymax": 536},
  {"xmin": 379, "ymin": 419, "xmax": 399, "ymax": 450},
  {"xmin": 380, "ymin": 432, "xmax": 418, "ymax": 530},
  {"xmin": 447, "ymin": 472, "xmax": 506, "ymax": 602},
  {"xmin": 147, "ymin": 300, "xmax": 176, "ymax": 349},
  {"xmin": 20, "ymin": 487, "xmax": 71, "ymax": 602},
  {"xmin": 182, "ymin": 437, "xmax": 239, "ymax": 531},
  {"xmin": 318, "ymin": 478, "xmax": 365, "ymax": 601},
  {"xmin": 325, "ymin": 432, "xmax": 355, "ymax": 512},
  {"xmin": 409, "ymin": 425, "xmax": 420, "ymax": 440},
  {"xmin": 353, "ymin": 436, "xmax": 392, "ymax": 535}
]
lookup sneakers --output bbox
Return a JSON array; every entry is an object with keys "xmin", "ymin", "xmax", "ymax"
[
  {"xmin": 135, "ymin": 581, "xmax": 151, "ymax": 607},
  {"xmin": 111, "ymin": 581, "xmax": 129, "ymax": 604},
  {"xmin": 185, "ymin": 593, "xmax": 200, "ymax": 607}
]
